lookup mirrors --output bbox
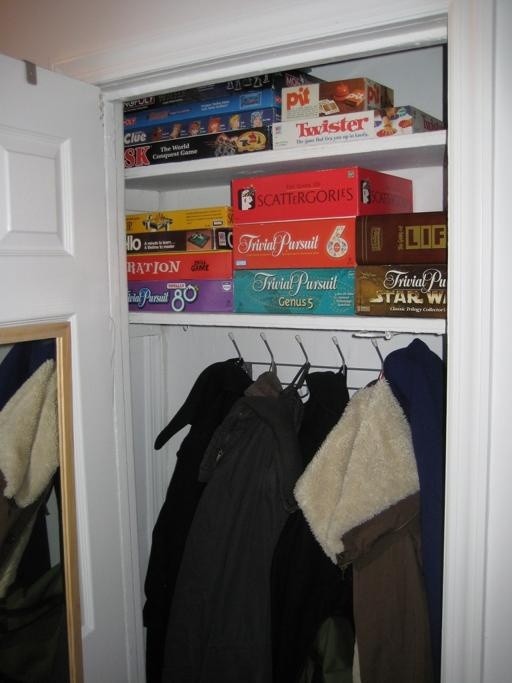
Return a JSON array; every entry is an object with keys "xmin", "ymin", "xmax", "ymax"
[{"xmin": 0, "ymin": 321, "xmax": 83, "ymax": 682}]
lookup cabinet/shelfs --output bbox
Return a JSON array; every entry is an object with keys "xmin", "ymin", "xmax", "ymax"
[{"xmin": 102, "ymin": 8, "xmax": 449, "ymax": 683}]
